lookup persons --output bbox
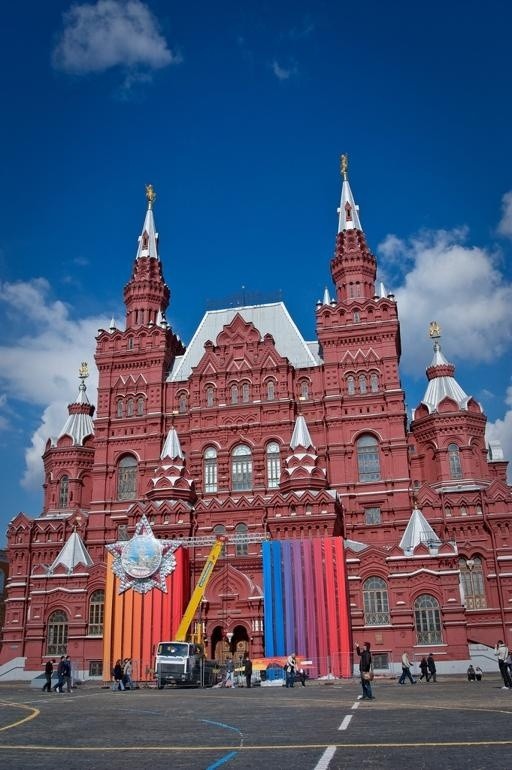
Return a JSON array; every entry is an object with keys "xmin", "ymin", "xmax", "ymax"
[
  {"xmin": 285, "ymin": 653, "xmax": 297, "ymax": 689},
  {"xmin": 427, "ymin": 653, "xmax": 437, "ymax": 683},
  {"xmin": 397, "ymin": 651, "xmax": 418, "ymax": 685},
  {"xmin": 225, "ymin": 658, "xmax": 237, "ymax": 689},
  {"xmin": 53, "ymin": 655, "xmax": 68, "ymax": 693},
  {"xmin": 41, "ymin": 659, "xmax": 57, "ymax": 692},
  {"xmin": 62, "ymin": 656, "xmax": 71, "ymax": 692},
  {"xmin": 419, "ymin": 656, "xmax": 429, "ymax": 682},
  {"xmin": 244, "ymin": 657, "xmax": 252, "ymax": 688},
  {"xmin": 494, "ymin": 640, "xmax": 512, "ymax": 690},
  {"xmin": 467, "ymin": 665, "xmax": 475, "ymax": 680},
  {"xmin": 113, "ymin": 657, "xmax": 136, "ymax": 691},
  {"xmin": 355, "ymin": 640, "xmax": 374, "ymax": 700},
  {"xmin": 476, "ymin": 667, "xmax": 482, "ymax": 681}
]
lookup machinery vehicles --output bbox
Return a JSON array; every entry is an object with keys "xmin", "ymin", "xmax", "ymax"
[{"xmin": 156, "ymin": 535, "xmax": 231, "ymax": 688}]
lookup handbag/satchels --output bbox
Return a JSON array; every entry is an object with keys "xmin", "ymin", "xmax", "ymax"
[
  {"xmin": 362, "ymin": 672, "xmax": 374, "ymax": 680},
  {"xmin": 283, "ymin": 665, "xmax": 288, "ymax": 671}
]
